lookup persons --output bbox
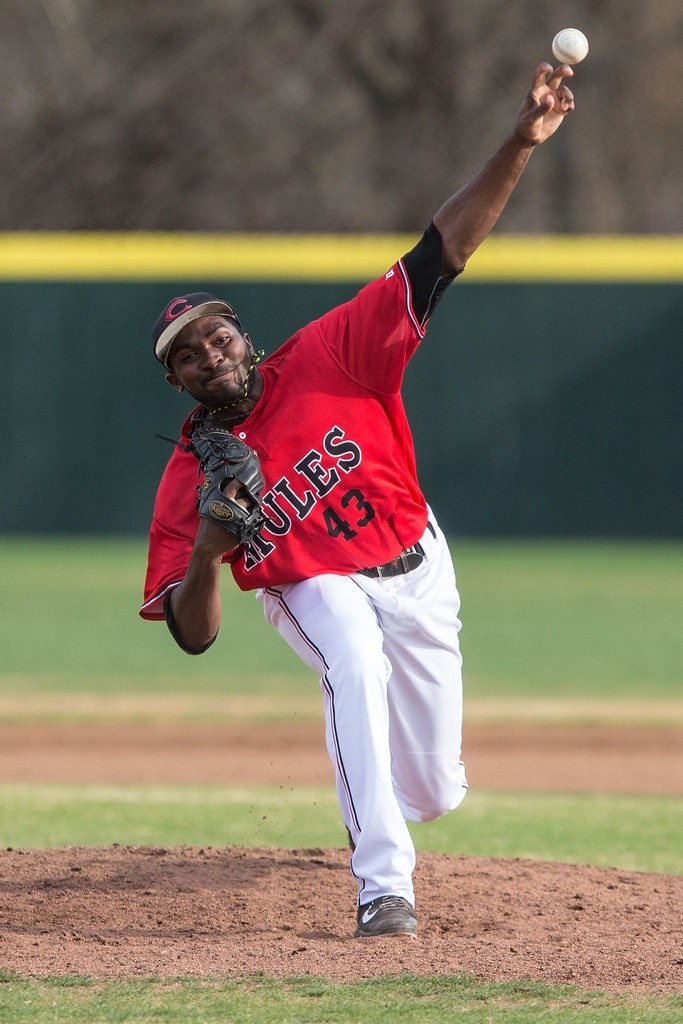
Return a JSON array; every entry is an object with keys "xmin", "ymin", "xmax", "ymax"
[{"xmin": 138, "ymin": 62, "xmax": 620, "ymax": 939}]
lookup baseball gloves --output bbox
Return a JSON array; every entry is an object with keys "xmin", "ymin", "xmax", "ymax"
[{"xmin": 189, "ymin": 427, "xmax": 265, "ymax": 547}]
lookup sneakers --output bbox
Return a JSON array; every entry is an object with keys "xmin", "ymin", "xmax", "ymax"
[{"xmin": 353, "ymin": 896, "xmax": 417, "ymax": 938}]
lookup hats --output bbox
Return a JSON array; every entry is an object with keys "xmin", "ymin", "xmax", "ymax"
[{"xmin": 152, "ymin": 292, "xmax": 242, "ymax": 373}]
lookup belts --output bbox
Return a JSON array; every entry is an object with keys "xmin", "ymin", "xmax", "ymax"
[{"xmin": 356, "ymin": 521, "xmax": 436, "ymax": 581}]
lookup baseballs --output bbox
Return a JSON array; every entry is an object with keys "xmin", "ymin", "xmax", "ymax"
[{"xmin": 552, "ymin": 28, "xmax": 589, "ymax": 64}]
